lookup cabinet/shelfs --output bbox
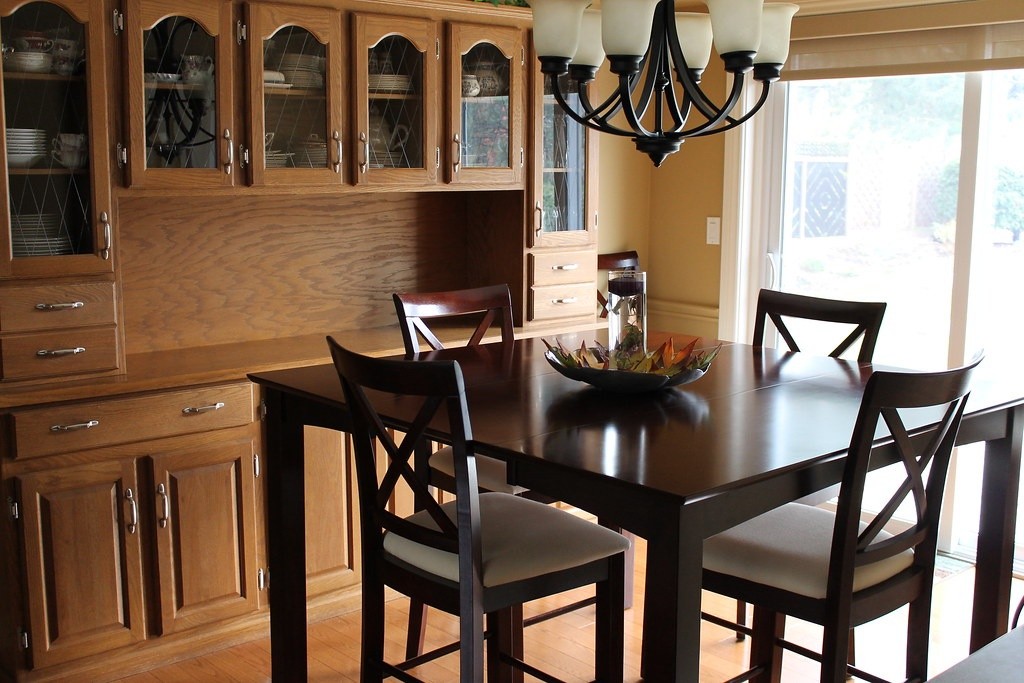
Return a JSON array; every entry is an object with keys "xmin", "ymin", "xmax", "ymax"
[{"xmin": 1, "ymin": 0, "xmax": 608, "ymax": 682}]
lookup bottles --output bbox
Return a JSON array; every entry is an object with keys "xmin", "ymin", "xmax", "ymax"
[{"xmin": 462, "ymin": 74, "xmax": 481, "ymax": 97}]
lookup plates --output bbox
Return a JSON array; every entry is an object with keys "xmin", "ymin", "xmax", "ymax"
[
  {"xmin": 246, "ymin": 52, "xmax": 415, "ymax": 169},
  {"xmin": 144, "ymin": 73, "xmax": 182, "ymax": 83},
  {"xmin": 2, "ymin": 128, "xmax": 88, "ymax": 256}
]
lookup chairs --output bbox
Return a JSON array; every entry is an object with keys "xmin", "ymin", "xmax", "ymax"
[
  {"xmin": 700, "ymin": 288, "xmax": 887, "ymax": 683},
  {"xmin": 698, "ymin": 349, "xmax": 986, "ymax": 682},
  {"xmin": 596, "ymin": 252, "xmax": 642, "ymax": 316},
  {"xmin": 325, "ymin": 335, "xmax": 631, "ymax": 683},
  {"xmin": 394, "ymin": 285, "xmax": 620, "ymax": 683}
]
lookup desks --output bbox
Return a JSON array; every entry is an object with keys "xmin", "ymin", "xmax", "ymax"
[{"xmin": 245, "ymin": 330, "xmax": 1024, "ymax": 682}]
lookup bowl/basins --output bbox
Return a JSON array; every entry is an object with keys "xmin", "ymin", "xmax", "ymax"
[{"xmin": 6, "ymin": 51, "xmax": 53, "ymax": 74}]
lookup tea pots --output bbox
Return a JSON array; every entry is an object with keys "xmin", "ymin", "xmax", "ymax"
[
  {"xmin": 461, "ymin": 58, "xmax": 513, "ymax": 99},
  {"xmin": 369, "ymin": 104, "xmax": 409, "ymax": 152}
]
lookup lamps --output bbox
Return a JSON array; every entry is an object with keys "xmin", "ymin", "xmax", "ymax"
[{"xmin": 522, "ymin": 0, "xmax": 800, "ymax": 167}]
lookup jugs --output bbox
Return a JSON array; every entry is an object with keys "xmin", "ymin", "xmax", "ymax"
[{"xmin": 180, "ymin": 54, "xmax": 214, "ymax": 86}]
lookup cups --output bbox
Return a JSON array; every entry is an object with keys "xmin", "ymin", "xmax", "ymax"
[
  {"xmin": 609, "ymin": 270, "xmax": 648, "ymax": 354},
  {"xmin": 51, "ymin": 150, "xmax": 86, "ymax": 169},
  {"xmin": 18, "ymin": 36, "xmax": 87, "ymax": 74},
  {"xmin": 52, "ymin": 133, "xmax": 85, "ymax": 151}
]
